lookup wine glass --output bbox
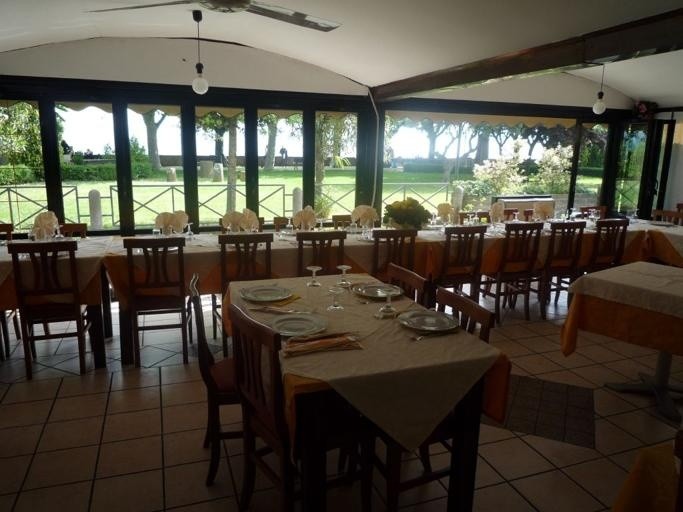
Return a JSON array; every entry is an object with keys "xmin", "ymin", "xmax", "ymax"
[
  {"xmin": 144, "ymin": 217, "xmax": 392, "ymax": 253},
  {"xmin": 379, "ymin": 287, "xmax": 398, "ymax": 321},
  {"xmin": 620, "ymin": 206, "xmax": 641, "ymax": 227},
  {"xmin": 303, "ymin": 264, "xmax": 352, "ymax": 316},
  {"xmin": 0, "ymin": 225, "xmax": 70, "ymax": 256},
  {"xmin": 426, "ymin": 208, "xmax": 605, "ymax": 238}
]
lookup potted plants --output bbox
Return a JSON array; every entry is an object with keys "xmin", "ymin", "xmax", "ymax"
[{"xmin": 382, "ymin": 195, "xmax": 434, "ymax": 237}]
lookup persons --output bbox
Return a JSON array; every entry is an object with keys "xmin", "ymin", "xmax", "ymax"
[
  {"xmin": 83, "ymin": 148, "xmax": 93, "ymax": 154},
  {"xmin": 385, "ymin": 146, "xmax": 396, "ymax": 169},
  {"xmin": 279, "ymin": 145, "xmax": 287, "ymax": 164}
]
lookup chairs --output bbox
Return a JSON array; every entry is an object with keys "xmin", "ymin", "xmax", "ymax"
[
  {"xmin": 188, "ymin": 273, "xmax": 279, "ymax": 487},
  {"xmin": 227, "ymin": 303, "xmax": 375, "ymax": 511},
  {"xmin": 299, "ymin": 256, "xmax": 497, "ymax": 508},
  {"xmin": 1, "ymin": 205, "xmax": 682, "ymax": 382}
]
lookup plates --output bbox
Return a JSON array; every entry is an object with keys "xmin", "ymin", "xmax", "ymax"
[
  {"xmin": 352, "ymin": 284, "xmax": 404, "ymax": 298},
  {"xmin": 398, "ymin": 309, "xmax": 462, "ymax": 333},
  {"xmin": 268, "ymin": 312, "xmax": 328, "ymax": 335},
  {"xmin": 238, "ymin": 285, "xmax": 292, "ymax": 302},
  {"xmin": 652, "ymin": 220, "xmax": 674, "ymax": 226}
]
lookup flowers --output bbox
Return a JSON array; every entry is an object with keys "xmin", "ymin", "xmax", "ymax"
[{"xmin": 630, "ymin": 95, "xmax": 660, "ymax": 123}]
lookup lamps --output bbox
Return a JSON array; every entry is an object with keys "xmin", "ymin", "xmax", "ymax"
[
  {"xmin": 80, "ymin": 0, "xmax": 344, "ymax": 34},
  {"xmin": 191, "ymin": 9, "xmax": 210, "ymax": 97},
  {"xmin": 580, "ymin": 61, "xmax": 607, "ymax": 116}
]
